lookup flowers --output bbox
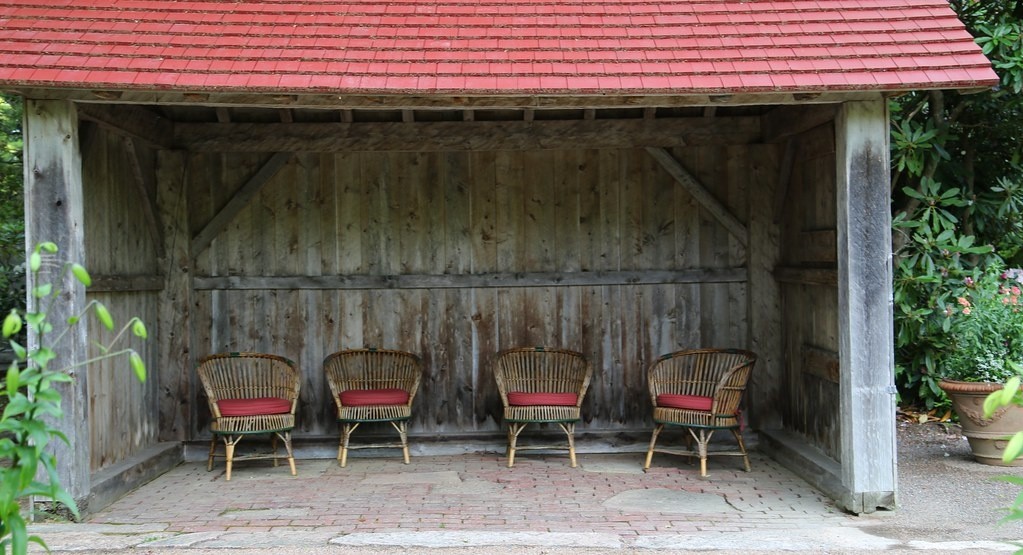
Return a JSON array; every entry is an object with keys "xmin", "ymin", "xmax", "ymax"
[{"xmin": 937, "ymin": 259, "xmax": 1023, "ymax": 384}]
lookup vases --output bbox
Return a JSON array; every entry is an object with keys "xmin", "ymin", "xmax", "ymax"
[{"xmin": 937, "ymin": 376, "xmax": 1022, "ymax": 468}]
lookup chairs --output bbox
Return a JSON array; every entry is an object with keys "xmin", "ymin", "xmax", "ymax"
[
  {"xmin": 323, "ymin": 348, "xmax": 425, "ymax": 467},
  {"xmin": 193, "ymin": 351, "xmax": 302, "ymax": 482},
  {"xmin": 644, "ymin": 347, "xmax": 759, "ymax": 478},
  {"xmin": 491, "ymin": 345, "xmax": 594, "ymax": 468}
]
